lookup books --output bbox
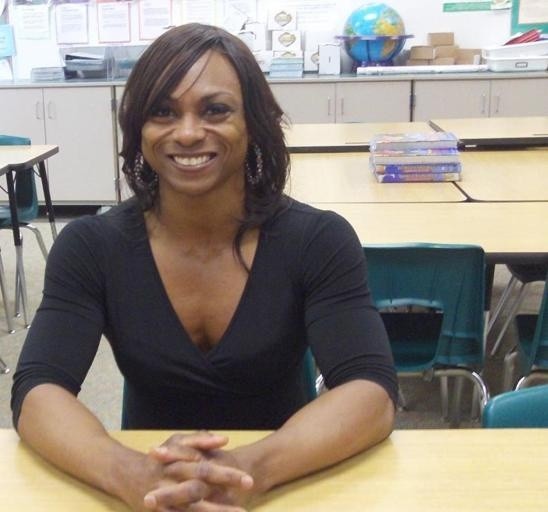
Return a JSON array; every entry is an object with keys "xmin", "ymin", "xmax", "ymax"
[
  {"xmin": 31, "ymin": 66, "xmax": 78, "ymax": 79},
  {"xmin": 369, "ymin": 130, "xmax": 462, "ymax": 182},
  {"xmin": 270, "ymin": 58, "xmax": 303, "ymax": 78}
]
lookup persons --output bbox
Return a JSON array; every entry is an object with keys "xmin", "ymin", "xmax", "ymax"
[{"xmin": 11, "ymin": 22, "xmax": 398, "ymax": 511}]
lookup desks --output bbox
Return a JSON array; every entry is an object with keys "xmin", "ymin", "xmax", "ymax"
[
  {"xmin": 1, "ymin": 142, "xmax": 61, "ymax": 376},
  {"xmin": 284, "ymin": 119, "xmax": 547, "ymax": 259},
  {"xmin": 3, "ymin": 426, "xmax": 548, "ymax": 509}
]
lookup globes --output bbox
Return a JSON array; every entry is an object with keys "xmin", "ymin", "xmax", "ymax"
[{"xmin": 335, "ymin": 3, "xmax": 416, "ymax": 74}]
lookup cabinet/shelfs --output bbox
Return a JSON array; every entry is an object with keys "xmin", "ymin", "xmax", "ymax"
[
  {"xmin": 268, "ymin": 77, "xmax": 411, "ymax": 122},
  {"xmin": 414, "ymin": 72, "xmax": 546, "ymax": 117},
  {"xmin": 2, "ymin": 82, "xmax": 120, "ymax": 212}
]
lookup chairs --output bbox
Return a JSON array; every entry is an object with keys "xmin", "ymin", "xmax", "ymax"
[
  {"xmin": 505, "ymin": 283, "xmax": 548, "ymax": 392},
  {"xmin": 325, "ymin": 239, "xmax": 490, "ymax": 429},
  {"xmin": 1, "ymin": 133, "xmax": 54, "ymax": 322},
  {"xmin": 485, "ymin": 385, "xmax": 546, "ymax": 429},
  {"xmin": 485, "ymin": 257, "xmax": 548, "ymax": 363}
]
